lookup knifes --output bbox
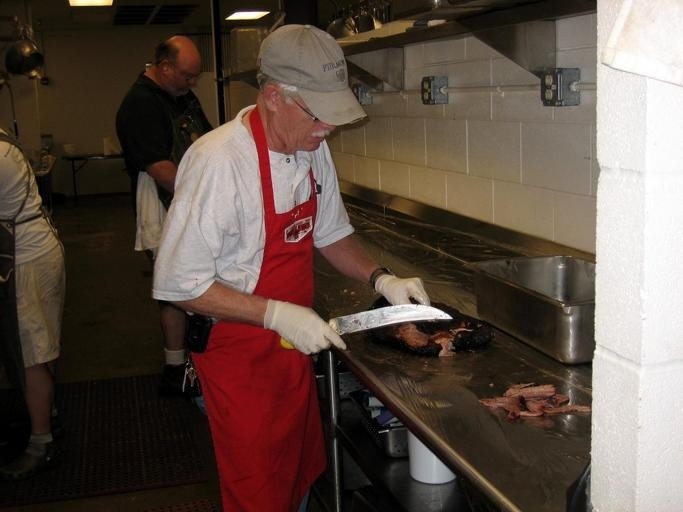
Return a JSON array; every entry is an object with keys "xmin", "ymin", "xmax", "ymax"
[{"xmin": 280, "ymin": 303, "xmax": 454, "ymax": 350}]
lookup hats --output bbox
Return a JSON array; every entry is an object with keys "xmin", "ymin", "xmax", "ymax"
[{"xmin": 255, "ymin": 22, "xmax": 367, "ymax": 129}]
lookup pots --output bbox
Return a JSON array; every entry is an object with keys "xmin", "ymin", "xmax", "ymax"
[{"xmin": 6, "ymin": 34, "xmax": 44, "ymax": 79}]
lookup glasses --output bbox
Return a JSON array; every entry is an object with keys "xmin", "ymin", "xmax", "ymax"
[{"xmin": 286, "ymin": 92, "xmax": 321, "ymax": 126}]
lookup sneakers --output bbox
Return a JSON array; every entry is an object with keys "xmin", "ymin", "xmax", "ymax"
[
  {"xmin": 161, "ymin": 357, "xmax": 193, "ymax": 389},
  {"xmin": 1, "ymin": 431, "xmax": 56, "ymax": 484}
]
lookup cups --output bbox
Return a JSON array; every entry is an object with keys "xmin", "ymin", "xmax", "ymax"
[{"xmin": 358, "ymin": 16, "xmax": 374, "ymax": 34}]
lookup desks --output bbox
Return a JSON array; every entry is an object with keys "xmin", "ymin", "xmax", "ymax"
[{"xmin": 61, "ymin": 153, "xmax": 126, "ymax": 207}]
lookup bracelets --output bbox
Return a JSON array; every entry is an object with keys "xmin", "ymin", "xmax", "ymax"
[{"xmin": 369, "ymin": 266, "xmax": 394, "ymax": 291}]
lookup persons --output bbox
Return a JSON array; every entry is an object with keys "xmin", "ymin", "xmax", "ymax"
[
  {"xmin": 151, "ymin": 22, "xmax": 429, "ymax": 509},
  {"xmin": 0, "ymin": 123, "xmax": 69, "ymax": 478},
  {"xmin": 113, "ymin": 35, "xmax": 214, "ymax": 399}
]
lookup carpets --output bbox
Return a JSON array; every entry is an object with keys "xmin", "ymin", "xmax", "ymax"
[
  {"xmin": 135, "ymin": 497, "xmax": 220, "ymax": 511},
  {"xmin": 0, "ymin": 373, "xmax": 218, "ymax": 508}
]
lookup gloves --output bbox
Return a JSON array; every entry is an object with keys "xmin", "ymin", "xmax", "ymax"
[
  {"xmin": 263, "ymin": 297, "xmax": 348, "ymax": 356},
  {"xmin": 374, "ymin": 273, "xmax": 435, "ymax": 323}
]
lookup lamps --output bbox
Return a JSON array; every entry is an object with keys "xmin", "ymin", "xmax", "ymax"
[{"xmin": 1, "ymin": 16, "xmax": 44, "ymax": 74}]
